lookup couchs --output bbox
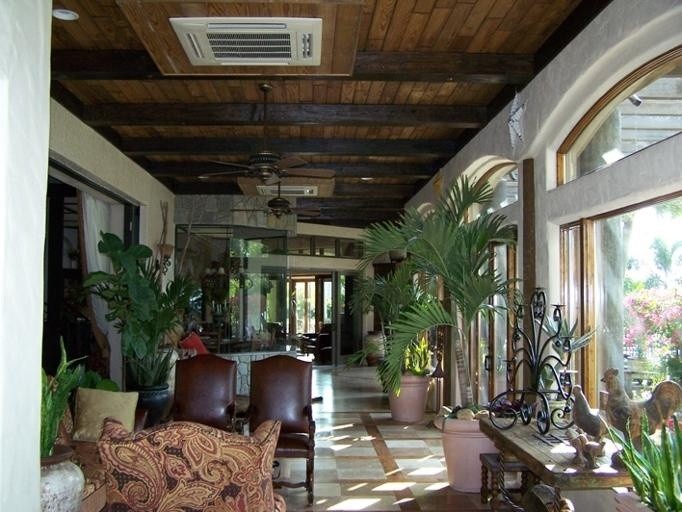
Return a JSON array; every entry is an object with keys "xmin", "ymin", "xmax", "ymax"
[{"xmin": 98, "ymin": 417, "xmax": 286, "ymax": 512}]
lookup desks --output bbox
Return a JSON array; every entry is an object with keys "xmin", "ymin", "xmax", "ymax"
[{"xmin": 479, "ymin": 416, "xmax": 634, "ymax": 512}]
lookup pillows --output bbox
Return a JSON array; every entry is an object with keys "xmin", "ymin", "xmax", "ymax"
[{"xmin": 72, "ymin": 387, "xmax": 139, "ymax": 443}]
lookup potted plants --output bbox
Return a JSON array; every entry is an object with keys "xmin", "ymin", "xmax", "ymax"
[
  {"xmin": 42, "ymin": 336, "xmax": 87, "ymax": 512},
  {"xmin": 357, "ymin": 176, "xmax": 516, "ymax": 493},
  {"xmin": 82, "ymin": 229, "xmax": 201, "ymax": 427},
  {"xmin": 341, "ymin": 258, "xmax": 432, "ymax": 423}
]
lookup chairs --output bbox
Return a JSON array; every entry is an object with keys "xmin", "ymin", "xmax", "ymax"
[
  {"xmin": 172, "ymin": 353, "xmax": 237, "ymax": 433},
  {"xmin": 235, "ymin": 357, "xmax": 314, "ymax": 507}
]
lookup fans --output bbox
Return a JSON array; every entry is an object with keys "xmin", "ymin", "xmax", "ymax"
[
  {"xmin": 199, "ymin": 85, "xmax": 333, "ymax": 181},
  {"xmin": 230, "ymin": 176, "xmax": 321, "ymax": 220}
]
